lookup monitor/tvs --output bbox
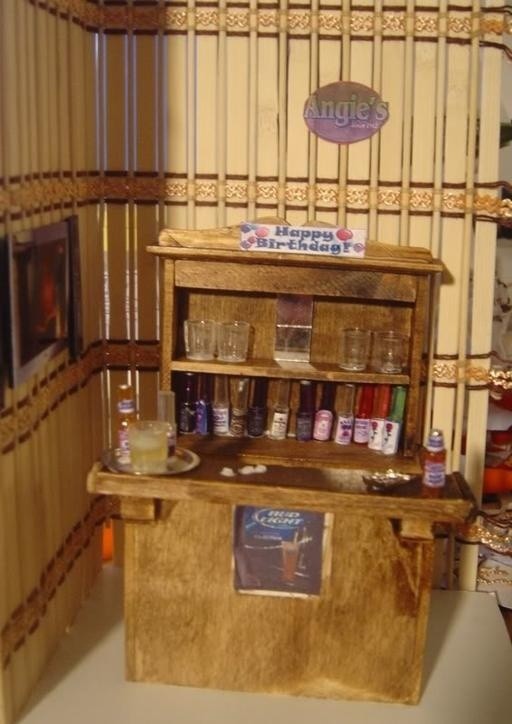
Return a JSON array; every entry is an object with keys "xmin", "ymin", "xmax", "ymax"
[{"xmin": 5, "ymin": 221, "xmax": 74, "ymax": 389}]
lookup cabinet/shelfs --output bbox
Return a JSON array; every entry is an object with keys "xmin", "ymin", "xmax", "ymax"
[{"xmin": 87, "ymin": 238, "xmax": 479, "ymax": 709}]
